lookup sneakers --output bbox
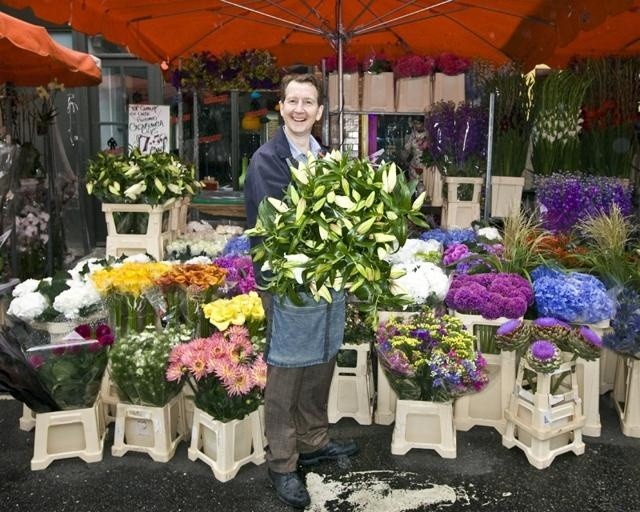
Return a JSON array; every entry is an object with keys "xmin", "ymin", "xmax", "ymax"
[
  {"xmin": 267, "ymin": 466, "xmax": 311, "ymax": 508},
  {"xmin": 298, "ymin": 436, "xmax": 359, "ymax": 465}
]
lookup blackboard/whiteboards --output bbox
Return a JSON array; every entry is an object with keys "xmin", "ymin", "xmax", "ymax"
[{"xmin": 127, "ymin": 104, "xmax": 170, "ymax": 155}]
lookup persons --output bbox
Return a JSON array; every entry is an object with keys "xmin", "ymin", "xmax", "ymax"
[
  {"xmin": 244, "ymin": 74, "xmax": 359, "ymax": 506},
  {"xmin": 401, "ymin": 115, "xmax": 430, "ymax": 180}
]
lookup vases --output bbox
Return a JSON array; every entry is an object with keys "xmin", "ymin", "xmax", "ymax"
[
  {"xmin": 187, "ymin": 407, "xmax": 267, "ymax": 483},
  {"xmin": 390, "ymin": 397, "xmax": 457, "ymax": 460},
  {"xmin": 31, "ymin": 392, "xmax": 108, "ymax": 471},
  {"xmin": 111, "ymin": 392, "xmax": 190, "ymax": 463},
  {"xmin": 502, "ymin": 394, "xmax": 586, "ymax": 470}
]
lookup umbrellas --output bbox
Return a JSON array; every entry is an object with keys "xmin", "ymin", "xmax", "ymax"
[
  {"xmin": 1, "ymin": 10, "xmax": 103, "ymax": 92},
  {"xmin": 542, "ymin": 0, "xmax": 639, "ymax": 71},
  {"xmin": 0, "ymin": 0, "xmax": 640, "ymax": 158}
]
